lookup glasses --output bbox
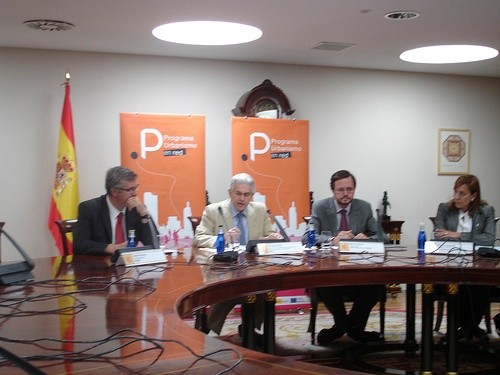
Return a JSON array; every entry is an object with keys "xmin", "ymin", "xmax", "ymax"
[{"xmin": 110, "ymin": 184, "xmax": 141, "ymax": 193}]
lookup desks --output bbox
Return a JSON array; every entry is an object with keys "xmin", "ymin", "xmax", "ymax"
[{"xmin": 1, "ymin": 241, "xmax": 500, "ymax": 375}]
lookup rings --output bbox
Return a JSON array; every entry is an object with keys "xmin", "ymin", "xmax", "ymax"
[{"xmin": 445, "ymin": 233, "xmax": 447, "ymax": 234}]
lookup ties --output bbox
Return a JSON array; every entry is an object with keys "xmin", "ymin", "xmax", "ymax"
[
  {"xmin": 236, "ymin": 213, "xmax": 246, "ymax": 245},
  {"xmin": 338, "ymin": 210, "xmax": 348, "ymax": 231},
  {"xmin": 116, "ymin": 212, "xmax": 124, "ymax": 244}
]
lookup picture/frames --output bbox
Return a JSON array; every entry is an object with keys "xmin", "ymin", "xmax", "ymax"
[{"xmin": 438, "ymin": 128, "xmax": 470, "ymax": 175}]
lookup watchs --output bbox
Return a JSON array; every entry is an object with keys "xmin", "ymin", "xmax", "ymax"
[{"xmin": 138, "ymin": 213, "xmax": 151, "ymax": 221}]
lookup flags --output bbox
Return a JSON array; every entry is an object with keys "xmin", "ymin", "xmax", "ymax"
[{"xmin": 48, "ymin": 83, "xmax": 79, "ymax": 258}]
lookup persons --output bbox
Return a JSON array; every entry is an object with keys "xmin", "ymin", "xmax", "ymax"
[
  {"xmin": 433, "ymin": 175, "xmax": 495, "ymax": 345},
  {"xmin": 195, "ymin": 172, "xmax": 284, "ymax": 345},
  {"xmin": 71, "ymin": 166, "xmax": 160, "ymax": 253},
  {"xmin": 301, "ymin": 170, "xmax": 384, "ymax": 344}
]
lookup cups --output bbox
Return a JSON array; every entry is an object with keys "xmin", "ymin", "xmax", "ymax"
[
  {"xmin": 228, "ymin": 231, "xmax": 242, "ymax": 251},
  {"xmin": 320, "ymin": 231, "xmax": 333, "ymax": 249}
]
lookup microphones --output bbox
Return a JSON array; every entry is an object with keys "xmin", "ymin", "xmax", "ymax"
[
  {"xmin": 462, "ymin": 216, "xmax": 464, "ymax": 220},
  {"xmin": 477, "ymin": 219, "xmax": 500, "ymax": 257},
  {"xmin": 212, "ymin": 207, "xmax": 238, "ymax": 262},
  {"xmin": 375, "ymin": 208, "xmax": 384, "ymax": 242},
  {"xmin": 147, "ymin": 216, "xmax": 158, "ymax": 249},
  {"xmin": 267, "ymin": 209, "xmax": 289, "ymax": 242}
]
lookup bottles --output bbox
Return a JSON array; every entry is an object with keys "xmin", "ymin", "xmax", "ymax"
[
  {"xmin": 418, "ymin": 222, "xmax": 426, "ymax": 255},
  {"xmin": 305, "ymin": 222, "xmax": 315, "ymax": 248},
  {"xmin": 216, "ymin": 224, "xmax": 225, "ymax": 254},
  {"xmin": 127, "ymin": 229, "xmax": 136, "ymax": 247}
]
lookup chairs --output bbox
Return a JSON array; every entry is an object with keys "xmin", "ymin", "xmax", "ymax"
[
  {"xmin": 55, "ymin": 219, "xmax": 77, "ymax": 255},
  {"xmin": 429, "ymin": 216, "xmax": 500, "ymax": 334},
  {"xmin": 303, "ymin": 216, "xmax": 385, "ymax": 345}
]
